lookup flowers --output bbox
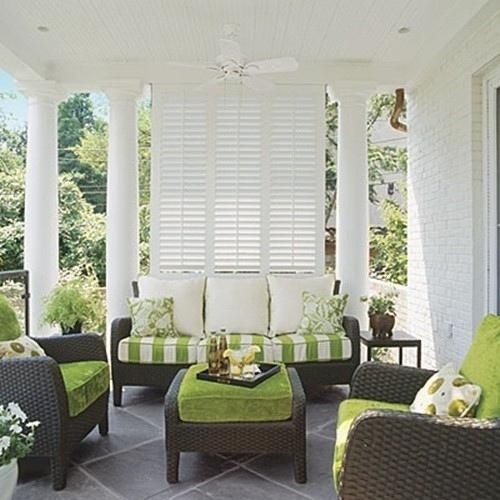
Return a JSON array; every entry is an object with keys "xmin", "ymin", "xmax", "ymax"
[{"xmin": 0, "ymin": 399, "xmax": 42, "ymax": 466}]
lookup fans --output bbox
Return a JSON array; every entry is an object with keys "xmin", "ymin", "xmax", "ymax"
[{"xmin": 173, "ymin": 24, "xmax": 297, "ymax": 96}]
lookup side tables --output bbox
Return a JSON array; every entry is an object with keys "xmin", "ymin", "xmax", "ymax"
[{"xmin": 359, "ymin": 330, "xmax": 421, "ymax": 368}]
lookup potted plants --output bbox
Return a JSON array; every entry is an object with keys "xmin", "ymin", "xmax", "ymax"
[
  {"xmin": 360, "ymin": 285, "xmax": 400, "ymax": 338},
  {"xmin": 38, "ymin": 277, "xmax": 104, "ymax": 335}
]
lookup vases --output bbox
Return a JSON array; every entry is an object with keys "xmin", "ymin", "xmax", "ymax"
[{"xmin": 0, "ymin": 458, "xmax": 19, "ymax": 500}]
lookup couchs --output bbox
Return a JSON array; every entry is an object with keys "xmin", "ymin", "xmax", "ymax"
[{"xmin": 110, "ymin": 280, "xmax": 361, "ymax": 407}]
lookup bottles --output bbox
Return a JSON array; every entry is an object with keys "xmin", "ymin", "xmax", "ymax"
[
  {"xmin": 219, "ymin": 327, "xmax": 230, "ymax": 375},
  {"xmin": 207, "ymin": 330, "xmax": 219, "ymax": 376}
]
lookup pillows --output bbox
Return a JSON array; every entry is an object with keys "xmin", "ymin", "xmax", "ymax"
[
  {"xmin": 0, "ymin": 334, "xmax": 46, "ymax": 357},
  {"xmin": 126, "ymin": 273, "xmax": 350, "ymax": 338},
  {"xmin": 408, "ymin": 361, "xmax": 483, "ymax": 419}
]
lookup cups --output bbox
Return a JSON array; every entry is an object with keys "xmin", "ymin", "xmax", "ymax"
[{"xmin": 243, "ymin": 362, "xmax": 255, "ymax": 379}]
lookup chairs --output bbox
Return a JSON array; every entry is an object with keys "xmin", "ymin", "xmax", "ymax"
[
  {"xmin": 332, "ymin": 311, "xmax": 500, "ymax": 498},
  {"xmin": 0, "ymin": 292, "xmax": 111, "ymax": 491}
]
